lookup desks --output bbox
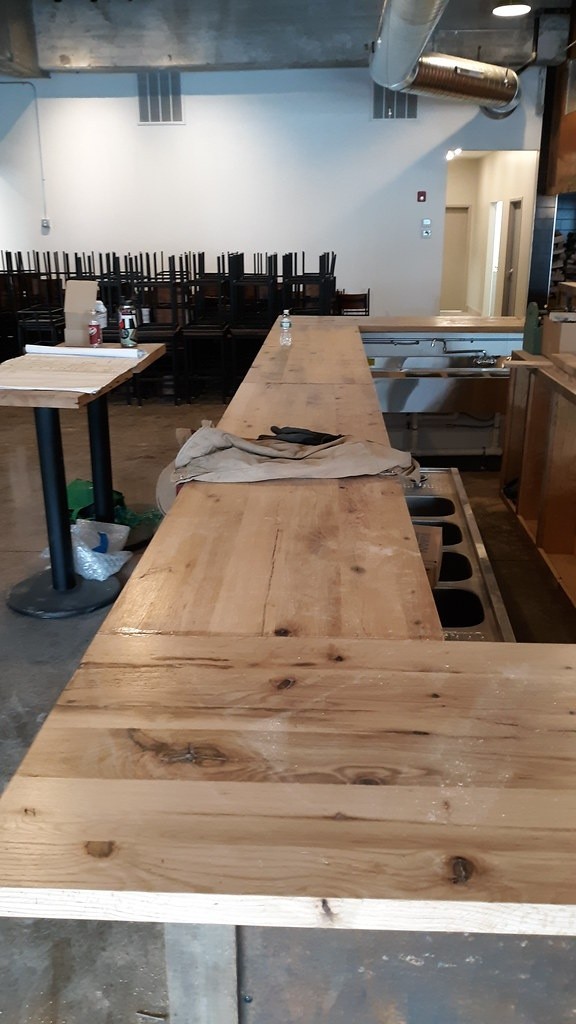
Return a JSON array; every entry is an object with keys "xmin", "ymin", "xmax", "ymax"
[
  {"xmin": 0, "ymin": 341, "xmax": 167, "ymax": 555},
  {"xmin": 0, "ymin": 356, "xmax": 134, "ymax": 620}
]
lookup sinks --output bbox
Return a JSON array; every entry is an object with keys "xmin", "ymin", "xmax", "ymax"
[
  {"xmin": 430, "ymin": 585, "xmax": 516, "ymax": 641},
  {"xmin": 410, "ymin": 519, "xmax": 485, "ymax": 550},
  {"xmin": 486, "ymin": 368, "xmax": 510, "ymax": 414},
  {"xmin": 404, "ymin": 494, "xmax": 476, "ymax": 519},
  {"xmin": 405, "ymin": 369, "xmax": 445, "ymax": 413},
  {"xmin": 418, "ymin": 550, "xmax": 498, "ymax": 586},
  {"xmin": 445, "ymin": 369, "xmax": 486, "ymax": 414},
  {"xmin": 365, "ymin": 369, "xmax": 405, "ymax": 414}
]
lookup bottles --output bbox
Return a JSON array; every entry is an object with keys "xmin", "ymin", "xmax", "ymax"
[
  {"xmin": 95, "ymin": 295, "xmax": 107, "ymax": 329},
  {"xmin": 89, "ymin": 308, "xmax": 102, "ymax": 348},
  {"xmin": 280, "ymin": 310, "xmax": 292, "ymax": 346},
  {"xmin": 118, "ymin": 300, "xmax": 137, "ymax": 348}
]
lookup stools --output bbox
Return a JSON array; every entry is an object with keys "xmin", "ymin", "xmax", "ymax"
[{"xmin": 0, "ymin": 249, "xmax": 370, "ymax": 408}]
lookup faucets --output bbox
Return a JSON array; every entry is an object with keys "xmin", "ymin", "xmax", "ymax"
[{"xmin": 431, "ymin": 338, "xmax": 447, "ymax": 353}]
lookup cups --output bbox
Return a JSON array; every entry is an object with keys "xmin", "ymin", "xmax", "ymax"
[{"xmin": 141, "ymin": 308, "xmax": 151, "ymax": 323}]
